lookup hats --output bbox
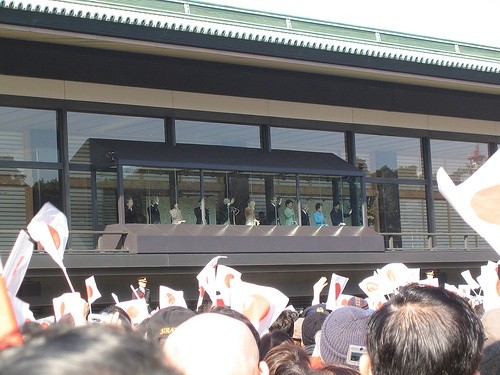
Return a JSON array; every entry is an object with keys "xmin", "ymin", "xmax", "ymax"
[
  {"xmin": 137, "ymin": 276, "xmax": 147, "ymax": 282},
  {"xmin": 146, "ymin": 306, "xmax": 197, "ymax": 347},
  {"xmin": 348, "ymin": 297, "xmax": 369, "ymax": 309},
  {"xmin": 301, "ymin": 312, "xmax": 326, "ymax": 344},
  {"xmin": 321, "ymin": 306, "xmax": 369, "ymax": 366},
  {"xmin": 304, "ymin": 303, "xmax": 330, "ymax": 316}
]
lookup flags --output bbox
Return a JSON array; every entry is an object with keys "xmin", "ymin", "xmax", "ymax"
[
  {"xmin": 436, "ymin": 147, "xmax": 500, "ymax": 257},
  {"xmin": 1, "ymin": 201, "xmax": 500, "ymax": 333}
]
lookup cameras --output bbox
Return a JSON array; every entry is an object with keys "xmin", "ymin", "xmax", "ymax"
[{"xmin": 346, "ymin": 345, "xmax": 367, "ymax": 366}]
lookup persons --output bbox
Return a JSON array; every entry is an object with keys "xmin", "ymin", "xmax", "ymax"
[
  {"xmin": 301, "ymin": 203, "xmax": 310, "ymax": 226},
  {"xmin": 267, "ymin": 197, "xmax": 282, "ymax": 225},
  {"xmin": 169, "ymin": 203, "xmax": 185, "ymax": 224},
  {"xmin": 258, "ymin": 211, "xmax": 266, "ymax": 225},
  {"xmin": 194, "ymin": 198, "xmax": 210, "ymax": 225},
  {"xmin": 124, "ymin": 197, "xmax": 139, "ymax": 224},
  {"xmin": 329, "ymin": 202, "xmax": 352, "ymax": 226},
  {"xmin": 1, "ymin": 276, "xmax": 500, "ymax": 374},
  {"xmin": 244, "ymin": 200, "xmax": 260, "ymax": 225},
  {"xmin": 147, "ymin": 196, "xmax": 161, "ymax": 224},
  {"xmin": 313, "ymin": 203, "xmax": 328, "ymax": 226},
  {"xmin": 284, "ymin": 199, "xmax": 298, "ymax": 225},
  {"xmin": 220, "ymin": 197, "xmax": 235, "ymax": 225}
]
